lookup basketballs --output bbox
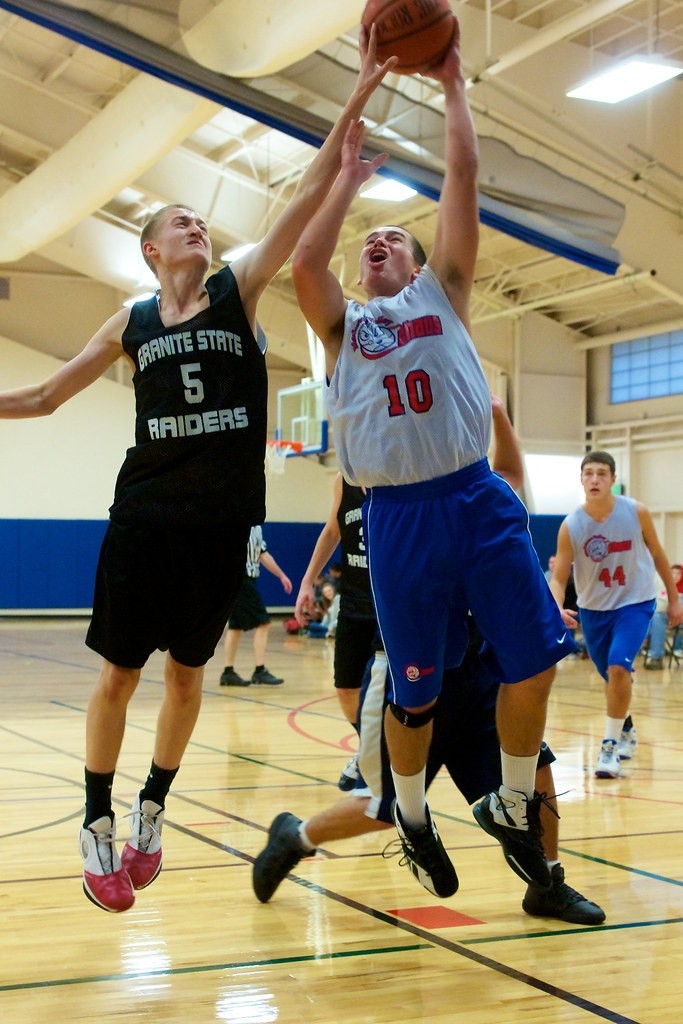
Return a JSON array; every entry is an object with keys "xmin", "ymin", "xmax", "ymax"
[{"xmin": 359, "ymin": 0, "xmax": 455, "ymax": 75}]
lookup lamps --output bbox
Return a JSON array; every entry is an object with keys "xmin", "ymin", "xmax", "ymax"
[
  {"xmin": 361, "ymin": 179, "xmax": 418, "ymax": 202},
  {"xmin": 565, "ymin": 52, "xmax": 683, "ymax": 104}
]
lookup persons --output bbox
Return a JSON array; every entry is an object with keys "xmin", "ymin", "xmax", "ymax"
[
  {"xmin": 219, "ymin": 393, "xmax": 606, "ymax": 926},
  {"xmin": 0, "ymin": 22, "xmax": 397, "ymax": 915},
  {"xmin": 544, "ymin": 453, "xmax": 683, "ymax": 780},
  {"xmin": 290, "ymin": 14, "xmax": 572, "ymax": 902}
]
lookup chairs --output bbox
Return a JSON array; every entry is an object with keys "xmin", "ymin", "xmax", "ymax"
[{"xmin": 645, "ymin": 622, "xmax": 683, "ymax": 670}]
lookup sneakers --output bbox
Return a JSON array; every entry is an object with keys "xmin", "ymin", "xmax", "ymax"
[
  {"xmin": 253, "ymin": 814, "xmax": 316, "ymax": 902},
  {"xmin": 251, "ymin": 669, "xmax": 284, "ymax": 685},
  {"xmin": 118, "ymin": 786, "xmax": 167, "ymax": 890},
  {"xmin": 620, "ymin": 726, "xmax": 636, "ymax": 757},
  {"xmin": 474, "ymin": 786, "xmax": 570, "ymax": 889},
  {"xmin": 79, "ymin": 819, "xmax": 135, "ymax": 913},
  {"xmin": 595, "ymin": 737, "xmax": 621, "ymax": 781},
  {"xmin": 382, "ymin": 794, "xmax": 459, "ymax": 898},
  {"xmin": 524, "ymin": 866, "xmax": 605, "ymax": 925},
  {"xmin": 221, "ymin": 670, "xmax": 250, "ymax": 687},
  {"xmin": 339, "ymin": 753, "xmax": 360, "ymax": 790}
]
